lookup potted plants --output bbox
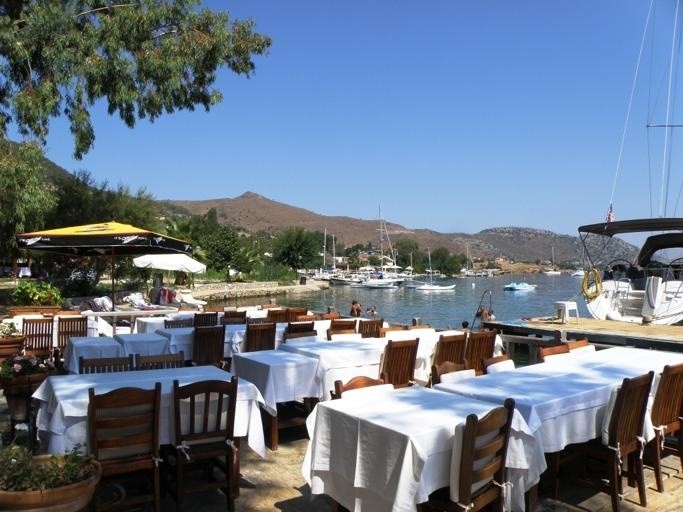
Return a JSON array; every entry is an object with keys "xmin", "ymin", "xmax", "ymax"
[
  {"xmin": 5, "ymin": 275, "xmax": 63, "ymax": 315},
  {"xmin": 0, "ymin": 321, "xmax": 25, "ymax": 356},
  {"xmin": 0, "ymin": 444, "xmax": 103, "ymax": 512}
]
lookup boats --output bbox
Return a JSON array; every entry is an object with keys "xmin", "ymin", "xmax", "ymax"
[
  {"xmin": 571, "ymin": 268, "xmax": 584, "ymax": 276},
  {"xmin": 417, "ymin": 284, "xmax": 457, "ymax": 290},
  {"xmin": 505, "ymin": 283, "xmax": 538, "ymax": 292}
]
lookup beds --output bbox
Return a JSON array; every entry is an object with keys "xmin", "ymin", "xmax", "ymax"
[
  {"xmin": 89, "ymin": 294, "xmax": 132, "ymax": 326},
  {"xmin": 175, "ymin": 289, "xmax": 207, "ymax": 311},
  {"xmin": 122, "ymin": 292, "xmax": 174, "ymax": 310}
]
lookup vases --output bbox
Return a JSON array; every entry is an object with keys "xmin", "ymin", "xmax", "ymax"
[{"xmin": 1, "ymin": 372, "xmax": 51, "ymax": 422}]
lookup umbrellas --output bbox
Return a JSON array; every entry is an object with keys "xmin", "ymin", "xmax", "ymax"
[
  {"xmin": 16, "ymin": 220, "xmax": 191, "ymax": 310},
  {"xmin": 132, "ymin": 253, "xmax": 206, "ymax": 303}
]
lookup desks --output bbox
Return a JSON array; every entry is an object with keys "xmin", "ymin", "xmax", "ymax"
[
  {"xmin": 308, "ymin": 386, "xmax": 531, "ymax": 511},
  {"xmin": 231, "ymin": 349, "xmax": 323, "ymax": 447},
  {"xmin": 135, "ymin": 306, "xmax": 313, "ymax": 331},
  {"xmin": 115, "ymin": 333, "xmax": 169, "ymax": 355},
  {"xmin": 156, "ymin": 317, "xmax": 387, "ymax": 361},
  {"xmin": 436, "ymin": 343, "xmax": 683, "ymax": 496},
  {"xmin": 281, "ymin": 330, "xmax": 503, "ymax": 393},
  {"xmin": 90, "ymin": 308, "xmax": 179, "ymax": 334},
  {"xmin": 3, "ymin": 318, "xmax": 97, "ymax": 346},
  {"xmin": 31, "ymin": 365, "xmax": 267, "ymax": 458},
  {"xmin": 63, "ymin": 336, "xmax": 121, "ymax": 364}
]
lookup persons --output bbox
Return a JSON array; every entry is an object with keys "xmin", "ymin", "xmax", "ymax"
[
  {"xmin": 365, "ymin": 307, "xmax": 371, "ymax": 314},
  {"xmin": 370, "ymin": 305, "xmax": 377, "ymax": 316},
  {"xmin": 462, "ymin": 321, "xmax": 469, "ymax": 332},
  {"xmin": 479, "ymin": 304, "xmax": 487, "ymax": 321},
  {"xmin": 174, "ymin": 275, "xmax": 186, "ymax": 285},
  {"xmin": 357, "ymin": 303, "xmax": 362, "ymax": 316},
  {"xmin": 487, "ymin": 309, "xmax": 496, "ymax": 321},
  {"xmin": 351, "ymin": 300, "xmax": 357, "ymax": 316}
]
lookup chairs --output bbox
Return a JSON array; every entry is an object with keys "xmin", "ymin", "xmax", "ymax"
[
  {"xmin": 568, "ymin": 340, "xmax": 597, "ymax": 361},
  {"xmin": 320, "ymin": 311, "xmax": 340, "ymax": 320},
  {"xmin": 359, "ymin": 320, "xmax": 383, "ymax": 340},
  {"xmin": 535, "ymin": 343, "xmax": 571, "ymax": 361},
  {"xmin": 330, "ymin": 375, "xmax": 390, "ymax": 397},
  {"xmin": 77, "ymin": 356, "xmax": 134, "ymax": 374},
  {"xmin": 485, "ymin": 353, "xmax": 516, "ymax": 372},
  {"xmin": 55, "ymin": 316, "xmax": 87, "ymax": 368},
  {"xmin": 283, "ymin": 321, "xmax": 318, "ymax": 340},
  {"xmin": 222, "ymin": 310, "xmax": 247, "ymax": 325},
  {"xmin": 329, "ymin": 318, "xmax": 358, "ymax": 329},
  {"xmin": 243, "ymin": 322, "xmax": 276, "ymax": 352},
  {"xmin": 161, "ymin": 375, "xmax": 242, "ymax": 510},
  {"xmin": 381, "ymin": 337, "xmax": 419, "ymax": 387},
  {"xmin": 135, "ymin": 350, "xmax": 187, "ymax": 370},
  {"xmin": 281, "ymin": 331, "xmax": 317, "ymax": 341},
  {"xmin": 22, "ymin": 317, "xmax": 53, "ymax": 357},
  {"xmin": 265, "ymin": 308, "xmax": 288, "ymax": 323},
  {"xmin": 193, "ymin": 312, "xmax": 218, "ymax": 326},
  {"xmin": 547, "ymin": 370, "xmax": 656, "ymax": 512},
  {"xmin": 190, "ymin": 324, "xmax": 229, "ymax": 370},
  {"xmin": 85, "ymin": 382, "xmax": 162, "ymax": 512},
  {"xmin": 645, "ymin": 365, "xmax": 682, "ymax": 492},
  {"xmin": 377, "ymin": 327, "xmax": 405, "ymax": 337},
  {"xmin": 430, "ymin": 358, "xmax": 470, "ymax": 382},
  {"xmin": 323, "ymin": 328, "xmax": 356, "ymax": 336},
  {"xmin": 417, "ymin": 398, "xmax": 520, "ymax": 512},
  {"xmin": 287, "ymin": 307, "xmax": 307, "ymax": 321},
  {"xmin": 295, "ymin": 315, "xmax": 317, "ymax": 321},
  {"xmin": 163, "ymin": 318, "xmax": 193, "ymax": 329},
  {"xmin": 406, "ymin": 324, "xmax": 431, "ymax": 333}
]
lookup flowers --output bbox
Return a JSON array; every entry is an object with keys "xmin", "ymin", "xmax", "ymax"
[{"xmin": 4, "ymin": 352, "xmax": 55, "ymax": 372}]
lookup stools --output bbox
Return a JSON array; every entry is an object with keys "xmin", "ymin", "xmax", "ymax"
[{"xmin": 551, "ymin": 301, "xmax": 579, "ymax": 325}]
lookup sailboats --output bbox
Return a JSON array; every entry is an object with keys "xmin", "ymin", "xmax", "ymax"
[
  {"xmin": 464, "ymin": 241, "xmax": 491, "ymax": 277},
  {"xmin": 579, "ymin": 1, "xmax": 682, "ymax": 326},
  {"xmin": 311, "ymin": 203, "xmax": 415, "ymax": 288},
  {"xmin": 544, "ymin": 247, "xmax": 561, "ymax": 275}
]
